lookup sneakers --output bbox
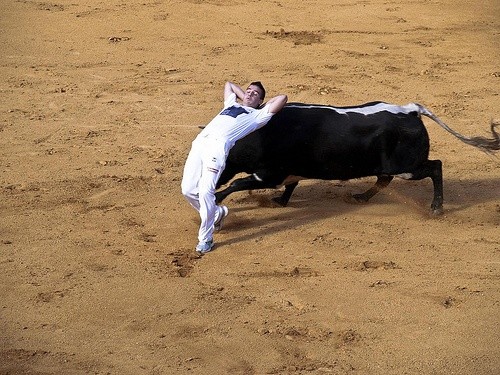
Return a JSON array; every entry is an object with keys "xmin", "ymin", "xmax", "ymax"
[
  {"xmin": 214, "ymin": 206, "xmax": 228, "ymax": 232},
  {"xmin": 195, "ymin": 239, "xmax": 214, "ymax": 253}
]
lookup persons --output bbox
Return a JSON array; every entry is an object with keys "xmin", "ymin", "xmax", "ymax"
[{"xmin": 180, "ymin": 80, "xmax": 289, "ymax": 254}]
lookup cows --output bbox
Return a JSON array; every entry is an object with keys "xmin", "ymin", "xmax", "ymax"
[{"xmin": 197, "ymin": 101, "xmax": 500, "ymax": 217}]
